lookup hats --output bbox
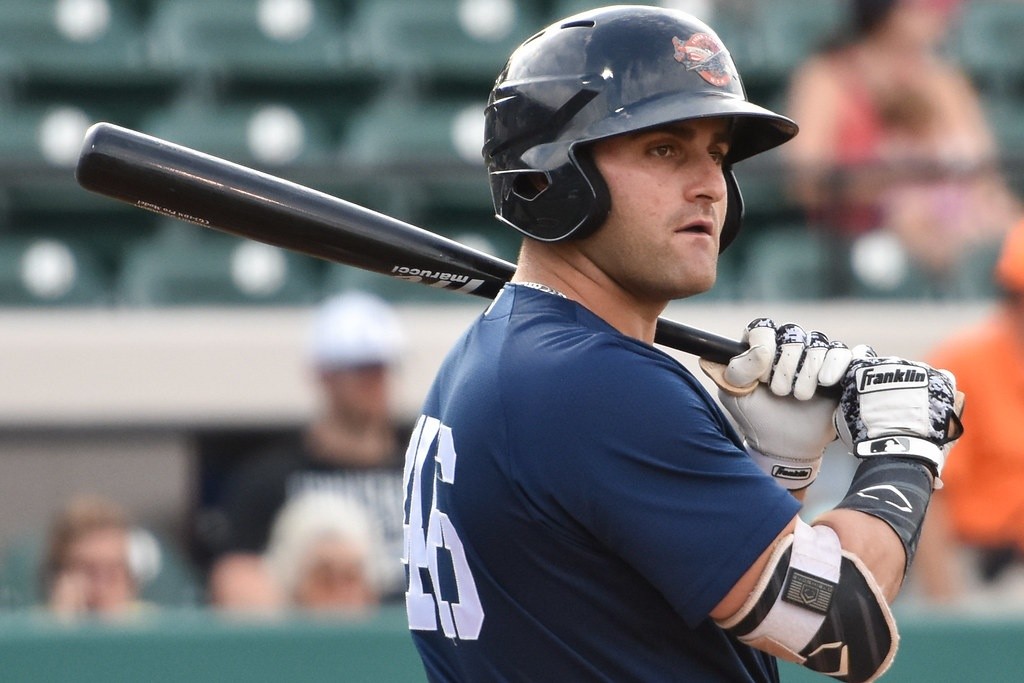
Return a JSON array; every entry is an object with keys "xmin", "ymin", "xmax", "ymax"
[{"xmin": 309, "ymin": 293, "xmax": 400, "ymax": 367}]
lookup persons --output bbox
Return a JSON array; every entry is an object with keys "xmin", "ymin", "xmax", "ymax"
[
  {"xmin": 42, "ymin": 286, "xmax": 429, "ymax": 620},
  {"xmin": 401, "ymin": 2, "xmax": 955, "ymax": 683},
  {"xmin": 910, "ymin": 214, "xmax": 1023, "ymax": 607},
  {"xmin": 775, "ymin": 0, "xmax": 1008, "ymax": 289}
]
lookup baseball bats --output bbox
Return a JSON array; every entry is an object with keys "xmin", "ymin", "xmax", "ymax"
[{"xmin": 74, "ymin": 119, "xmax": 967, "ymax": 417}]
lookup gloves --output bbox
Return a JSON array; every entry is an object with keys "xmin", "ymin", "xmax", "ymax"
[
  {"xmin": 698, "ymin": 318, "xmax": 852, "ymax": 489},
  {"xmin": 832, "ymin": 345, "xmax": 964, "ymax": 491}
]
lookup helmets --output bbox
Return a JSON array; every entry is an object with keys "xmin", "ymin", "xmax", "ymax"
[{"xmin": 481, "ymin": 6, "xmax": 799, "ymax": 255}]
